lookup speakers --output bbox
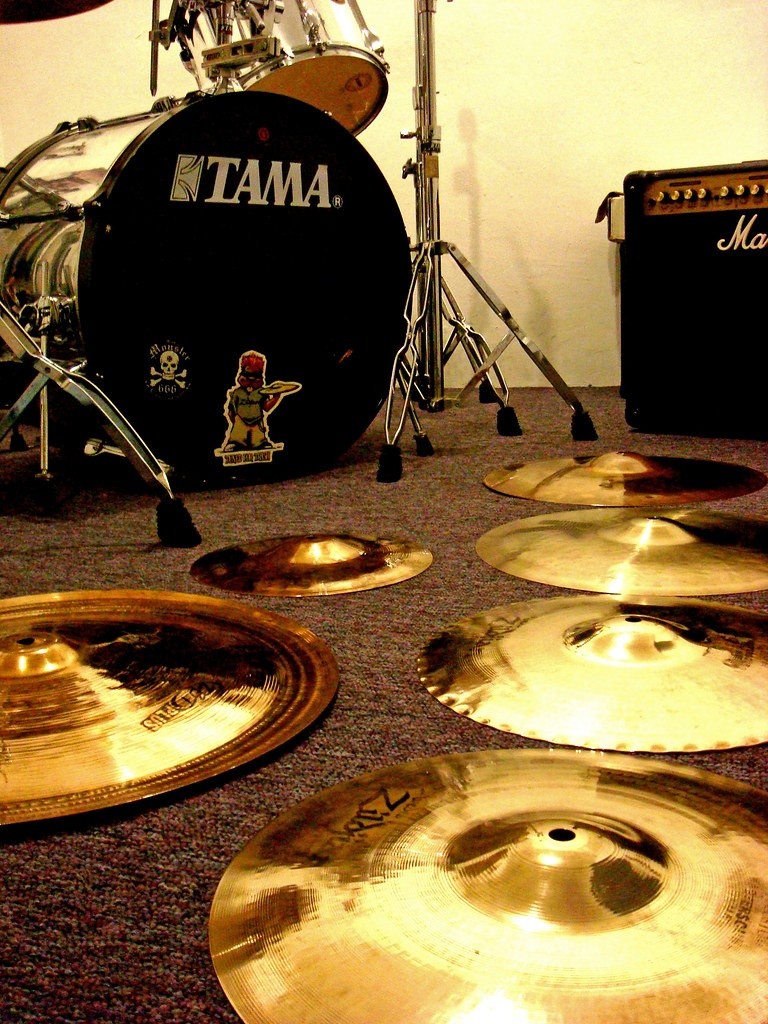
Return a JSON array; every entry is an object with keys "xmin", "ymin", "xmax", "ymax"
[{"xmin": 619, "ymin": 160, "xmax": 768, "ymax": 445}]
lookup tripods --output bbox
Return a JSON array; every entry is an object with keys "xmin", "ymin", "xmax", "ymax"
[{"xmin": 372, "ymin": 0, "xmax": 599, "ymax": 482}]
